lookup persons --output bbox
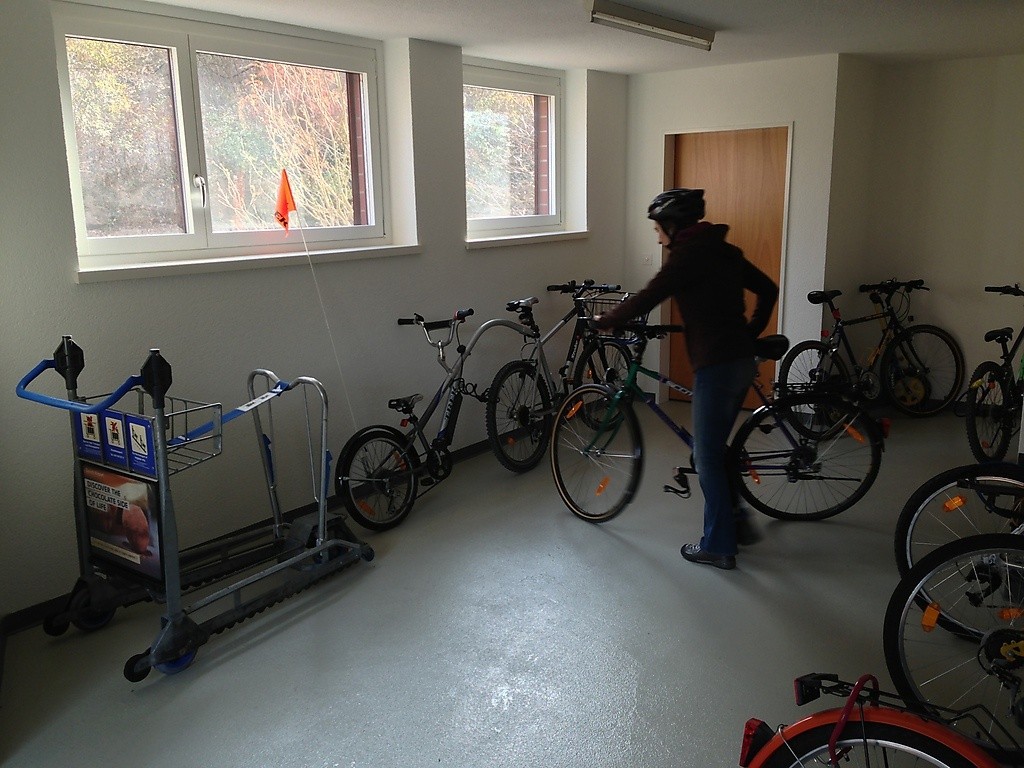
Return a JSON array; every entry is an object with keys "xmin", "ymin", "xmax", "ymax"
[{"xmin": 592, "ymin": 188, "xmax": 779, "ymax": 570}]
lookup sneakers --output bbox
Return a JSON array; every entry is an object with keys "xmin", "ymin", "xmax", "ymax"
[
  {"xmin": 681, "ymin": 543, "xmax": 735, "ymax": 570},
  {"xmin": 735, "ymin": 524, "xmax": 753, "ymax": 545}
]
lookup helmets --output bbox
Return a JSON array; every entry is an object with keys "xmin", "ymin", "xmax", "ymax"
[{"xmin": 647, "ymin": 187, "xmax": 705, "ymax": 226}]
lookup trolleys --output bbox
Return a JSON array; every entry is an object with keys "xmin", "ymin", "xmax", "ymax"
[{"xmin": 18, "ymin": 335, "xmax": 376, "ymax": 686}]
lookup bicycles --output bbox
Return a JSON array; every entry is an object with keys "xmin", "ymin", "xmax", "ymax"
[
  {"xmin": 332, "ymin": 278, "xmax": 651, "ymax": 534},
  {"xmin": 883, "ymin": 479, "xmax": 1023, "ymax": 768},
  {"xmin": 548, "ymin": 315, "xmax": 892, "ymax": 524},
  {"xmin": 955, "ymin": 282, "xmax": 1024, "ymax": 468},
  {"xmin": 738, "ymin": 671, "xmax": 1024, "ymax": 768},
  {"xmin": 777, "ymin": 278, "xmax": 964, "ymax": 441},
  {"xmin": 892, "ymin": 452, "xmax": 1024, "ymax": 646}
]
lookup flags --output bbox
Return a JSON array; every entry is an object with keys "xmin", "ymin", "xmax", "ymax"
[{"xmin": 274, "ymin": 170, "xmax": 296, "ymax": 239}]
